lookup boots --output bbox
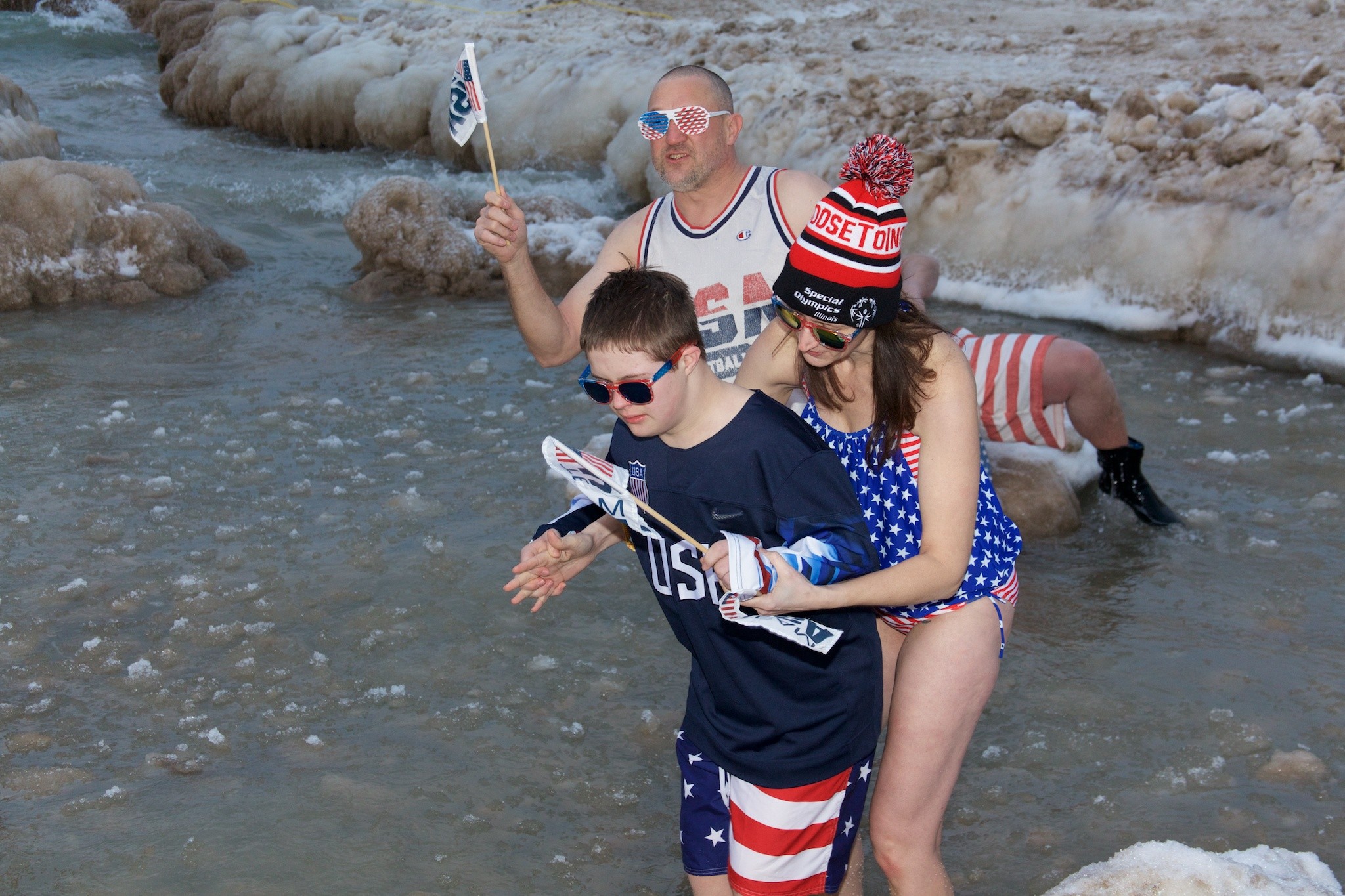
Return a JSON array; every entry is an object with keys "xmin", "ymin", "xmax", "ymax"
[{"xmin": 1096, "ymin": 438, "xmax": 1184, "ymax": 528}]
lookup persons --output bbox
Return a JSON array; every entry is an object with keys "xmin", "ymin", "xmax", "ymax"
[
  {"xmin": 502, "ymin": 134, "xmax": 1023, "ymax": 896},
  {"xmin": 500, "ymin": 267, "xmax": 884, "ymax": 896},
  {"xmin": 473, "ymin": 66, "xmax": 1192, "ymax": 534}
]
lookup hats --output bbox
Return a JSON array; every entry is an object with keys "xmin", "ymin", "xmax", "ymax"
[{"xmin": 772, "ymin": 132, "xmax": 913, "ymax": 328}]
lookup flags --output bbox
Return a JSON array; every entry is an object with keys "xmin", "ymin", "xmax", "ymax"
[
  {"xmin": 446, "ymin": 43, "xmax": 490, "ymax": 147},
  {"xmin": 541, "ymin": 434, "xmax": 663, "ymax": 540}
]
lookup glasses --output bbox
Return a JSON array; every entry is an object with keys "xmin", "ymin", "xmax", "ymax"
[
  {"xmin": 638, "ymin": 106, "xmax": 733, "ymax": 141},
  {"xmin": 772, "ymin": 297, "xmax": 861, "ymax": 351},
  {"xmin": 576, "ymin": 340, "xmax": 699, "ymax": 406}
]
